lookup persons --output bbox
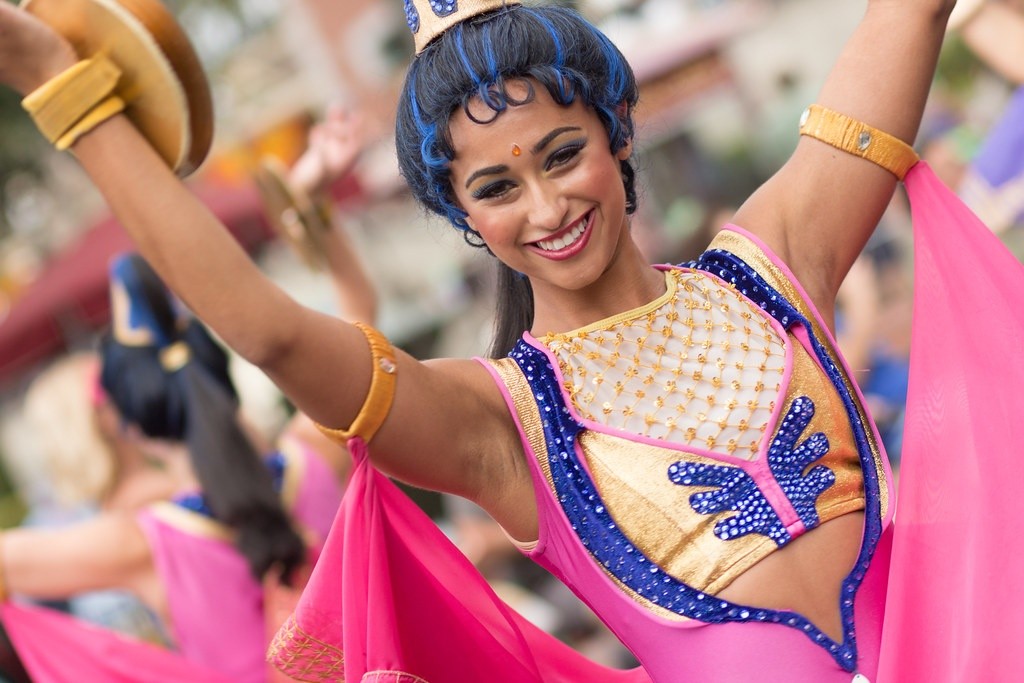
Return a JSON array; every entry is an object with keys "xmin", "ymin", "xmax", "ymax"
[{"xmin": 0, "ymin": 0, "xmax": 1024, "ymax": 683}]
